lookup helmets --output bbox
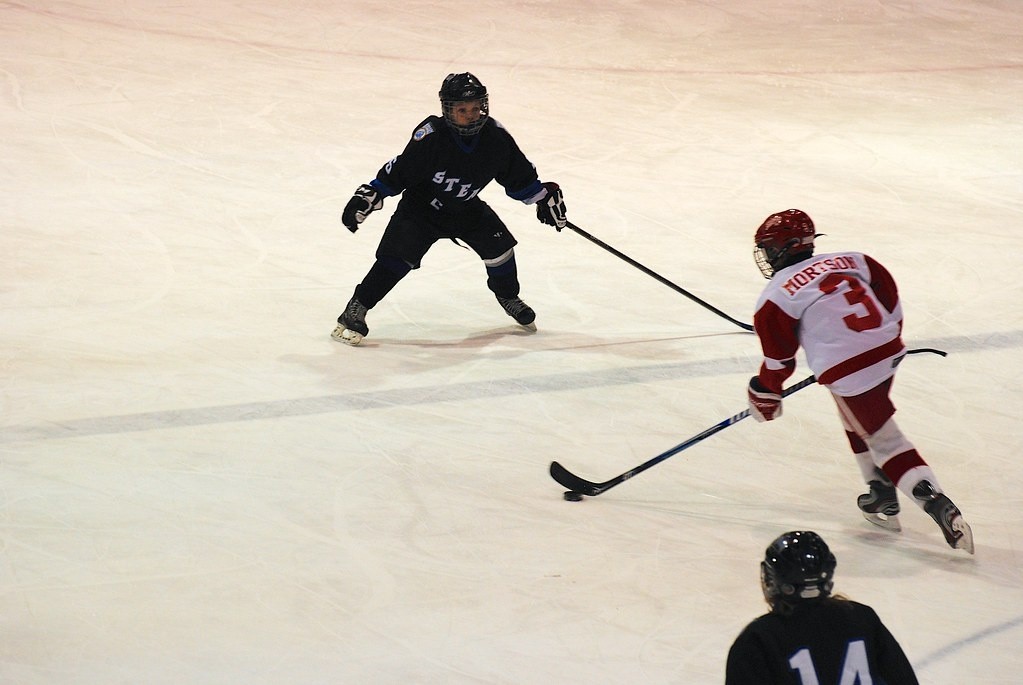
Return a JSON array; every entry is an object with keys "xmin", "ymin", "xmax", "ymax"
[
  {"xmin": 439, "ymin": 72, "xmax": 490, "ymax": 136},
  {"xmin": 753, "ymin": 209, "xmax": 814, "ymax": 280},
  {"xmin": 760, "ymin": 531, "xmax": 836, "ymax": 610}
]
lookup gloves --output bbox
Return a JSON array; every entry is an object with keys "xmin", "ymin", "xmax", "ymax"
[
  {"xmin": 342, "ymin": 185, "xmax": 383, "ymax": 233},
  {"xmin": 748, "ymin": 377, "xmax": 783, "ymax": 422},
  {"xmin": 534, "ymin": 182, "xmax": 567, "ymax": 231}
]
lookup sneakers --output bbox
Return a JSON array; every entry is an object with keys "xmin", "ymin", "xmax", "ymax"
[
  {"xmin": 912, "ymin": 481, "xmax": 974, "ymax": 554},
  {"xmin": 330, "ymin": 285, "xmax": 369, "ymax": 346},
  {"xmin": 858, "ymin": 467, "xmax": 901, "ymax": 533},
  {"xmin": 494, "ymin": 292, "xmax": 537, "ymax": 332}
]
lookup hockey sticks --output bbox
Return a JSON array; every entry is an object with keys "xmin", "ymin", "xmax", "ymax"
[
  {"xmin": 564, "ymin": 219, "xmax": 757, "ymax": 332},
  {"xmin": 548, "ymin": 374, "xmax": 820, "ymax": 498}
]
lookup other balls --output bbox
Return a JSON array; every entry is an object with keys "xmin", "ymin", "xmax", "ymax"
[{"xmin": 564, "ymin": 490, "xmax": 585, "ymax": 502}]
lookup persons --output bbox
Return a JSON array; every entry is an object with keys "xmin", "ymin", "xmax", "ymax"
[
  {"xmin": 724, "ymin": 529, "xmax": 921, "ymax": 684},
  {"xmin": 747, "ymin": 208, "xmax": 975, "ymax": 554},
  {"xmin": 331, "ymin": 72, "xmax": 565, "ymax": 344}
]
